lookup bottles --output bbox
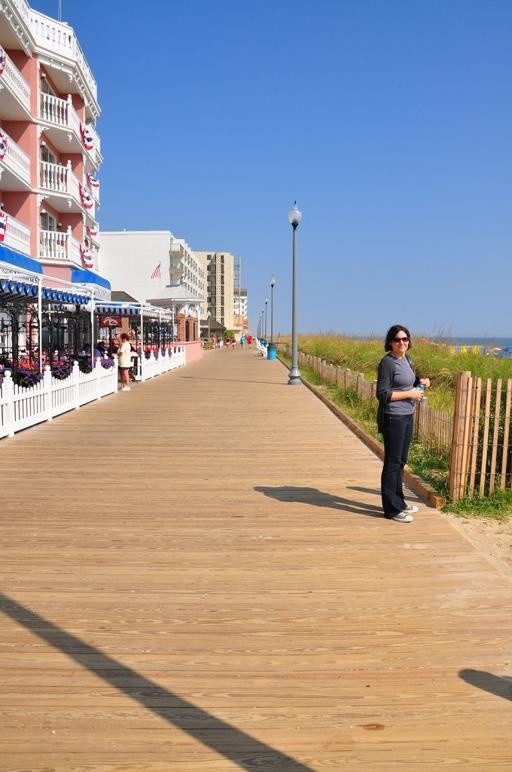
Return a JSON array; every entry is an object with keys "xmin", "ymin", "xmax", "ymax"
[{"xmin": 416, "ymin": 383, "xmax": 425, "ymax": 400}]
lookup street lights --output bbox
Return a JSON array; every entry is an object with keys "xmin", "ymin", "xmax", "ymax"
[
  {"xmin": 257, "ymin": 298, "xmax": 268, "ymax": 339},
  {"xmin": 288, "ymin": 200, "xmax": 302, "ymax": 386},
  {"xmin": 270, "ymin": 274, "xmax": 276, "ymax": 344}
]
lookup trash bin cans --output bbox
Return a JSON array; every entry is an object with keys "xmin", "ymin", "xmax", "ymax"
[
  {"xmin": 257, "ymin": 337, "xmax": 267, "ymax": 348},
  {"xmin": 267, "ymin": 343, "xmax": 277, "ymax": 360}
]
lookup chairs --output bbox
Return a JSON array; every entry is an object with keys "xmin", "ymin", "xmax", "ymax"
[{"xmin": 1, "ymin": 339, "xmax": 150, "ymax": 387}]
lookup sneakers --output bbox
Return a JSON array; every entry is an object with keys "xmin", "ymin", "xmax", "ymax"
[
  {"xmin": 119, "ymin": 384, "xmax": 132, "ymax": 392},
  {"xmin": 392, "ymin": 512, "xmax": 413, "ymax": 523},
  {"xmin": 402, "ymin": 505, "xmax": 418, "ymax": 513}
]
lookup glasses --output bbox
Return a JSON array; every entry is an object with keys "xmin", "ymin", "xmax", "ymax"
[{"xmin": 393, "ymin": 337, "xmax": 411, "ymax": 343}]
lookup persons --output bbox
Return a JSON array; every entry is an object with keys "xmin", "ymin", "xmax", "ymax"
[
  {"xmin": 375, "ymin": 324, "xmax": 430, "ymax": 522},
  {"xmin": 239, "ymin": 332, "xmax": 246, "ymax": 347},
  {"xmin": 116, "ymin": 332, "xmax": 131, "ymax": 392},
  {"xmin": 247, "ymin": 334, "xmax": 253, "ymax": 348}
]
